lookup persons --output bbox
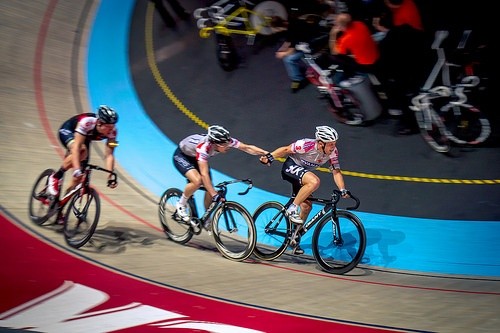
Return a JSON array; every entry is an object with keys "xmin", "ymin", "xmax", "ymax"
[
  {"xmin": 46, "ymin": 104, "xmax": 118, "ymax": 224},
  {"xmin": 173, "ymin": 126, "xmax": 274, "ymax": 232},
  {"xmin": 251, "ymin": 0, "xmax": 449, "ymax": 137},
  {"xmin": 260, "ymin": 125, "xmax": 351, "ymax": 254}
]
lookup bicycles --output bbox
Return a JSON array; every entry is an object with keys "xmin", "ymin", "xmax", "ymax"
[
  {"xmin": 28, "ymin": 159, "xmax": 118, "ymax": 248},
  {"xmin": 248, "ymin": 189, "xmax": 366, "ymax": 275},
  {"xmin": 158, "ymin": 177, "xmax": 257, "ymax": 262}
]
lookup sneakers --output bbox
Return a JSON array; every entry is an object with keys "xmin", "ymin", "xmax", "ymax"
[
  {"xmin": 177, "ymin": 203, "xmax": 190, "ymax": 221},
  {"xmin": 286, "ymin": 209, "xmax": 303, "ymax": 224},
  {"xmin": 289, "ymin": 240, "xmax": 304, "ymax": 255},
  {"xmin": 202, "ymin": 215, "xmax": 213, "ymax": 231},
  {"xmin": 56, "ymin": 211, "xmax": 64, "ymax": 225},
  {"xmin": 49, "ymin": 173, "xmax": 59, "ymax": 195}
]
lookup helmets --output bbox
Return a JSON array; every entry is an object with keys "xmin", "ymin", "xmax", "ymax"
[
  {"xmin": 207, "ymin": 125, "xmax": 230, "ymax": 145},
  {"xmin": 98, "ymin": 105, "xmax": 118, "ymax": 124},
  {"xmin": 316, "ymin": 125, "xmax": 338, "ymax": 143}
]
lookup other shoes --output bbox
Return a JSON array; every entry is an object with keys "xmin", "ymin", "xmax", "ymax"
[
  {"xmin": 397, "ymin": 127, "xmax": 419, "ymax": 135},
  {"xmin": 291, "ymin": 80, "xmax": 299, "ymax": 93}
]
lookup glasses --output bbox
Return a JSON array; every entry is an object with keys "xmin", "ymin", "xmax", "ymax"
[{"xmin": 214, "ymin": 142, "xmax": 229, "ymax": 148}]
